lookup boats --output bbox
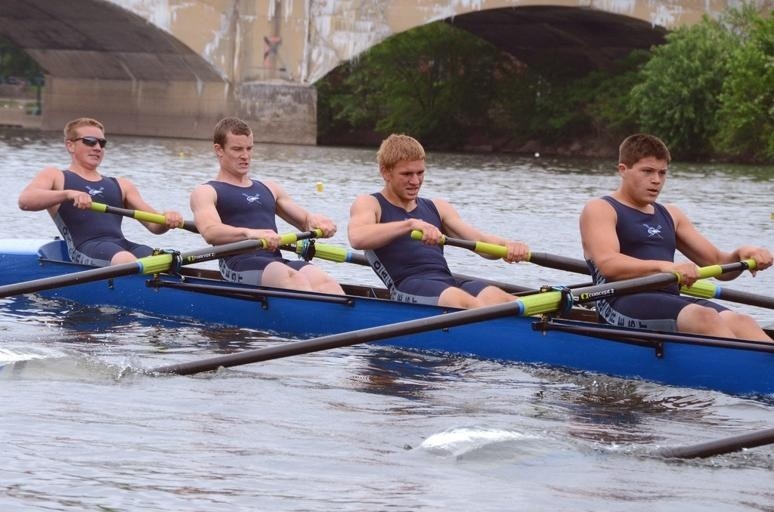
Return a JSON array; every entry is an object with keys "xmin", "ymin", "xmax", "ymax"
[{"xmin": 0, "ymin": 235, "xmax": 774, "ymax": 397}]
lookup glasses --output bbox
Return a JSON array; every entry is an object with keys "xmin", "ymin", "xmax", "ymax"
[{"xmin": 71, "ymin": 136, "xmax": 106, "ymax": 147}]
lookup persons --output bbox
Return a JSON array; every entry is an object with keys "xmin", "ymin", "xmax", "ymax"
[
  {"xmin": 580, "ymin": 133, "xmax": 774, "ymax": 344},
  {"xmin": 19, "ymin": 118, "xmax": 184, "ymax": 267},
  {"xmin": 190, "ymin": 117, "xmax": 347, "ymax": 295},
  {"xmin": 348, "ymin": 133, "xmax": 529, "ymax": 308}
]
